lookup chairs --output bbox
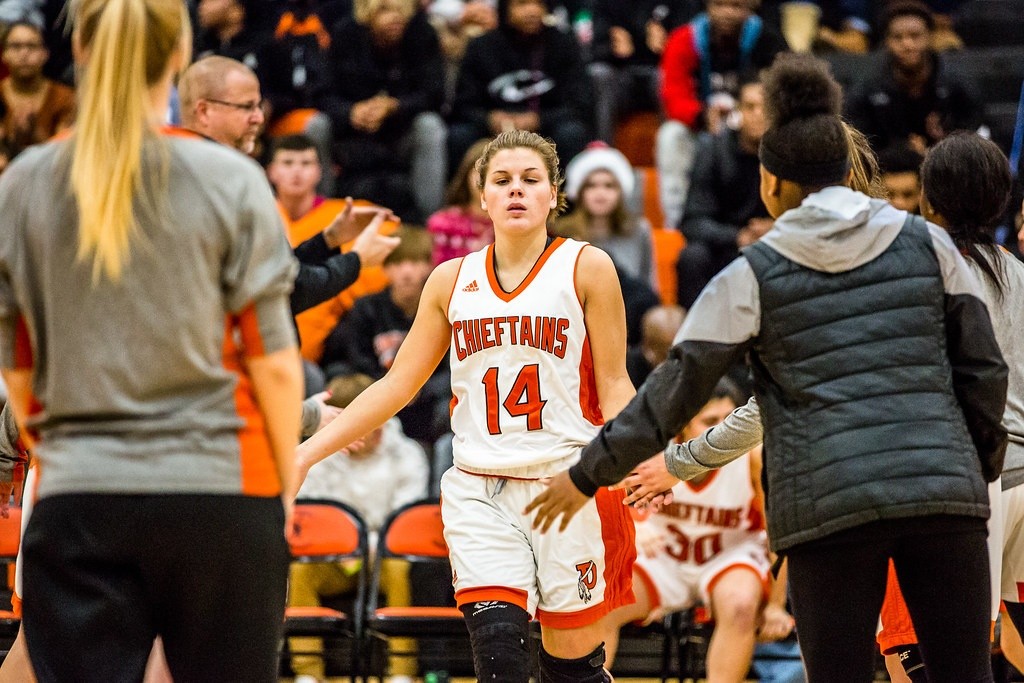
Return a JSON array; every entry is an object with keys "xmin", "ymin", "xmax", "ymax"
[
  {"xmin": 362, "ymin": 498, "xmax": 471, "ymax": 683},
  {"xmin": 619, "ymin": 604, "xmax": 796, "ymax": 682},
  {"xmin": 282, "ymin": 498, "xmax": 369, "ymax": 683}
]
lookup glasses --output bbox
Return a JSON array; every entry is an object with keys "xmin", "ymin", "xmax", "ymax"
[{"xmin": 208, "ymin": 99, "xmax": 263, "ymax": 111}]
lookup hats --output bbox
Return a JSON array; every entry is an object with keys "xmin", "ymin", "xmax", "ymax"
[{"xmin": 565, "ymin": 141, "xmax": 640, "ymax": 217}]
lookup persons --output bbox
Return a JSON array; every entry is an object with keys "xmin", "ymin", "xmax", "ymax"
[{"xmin": 1, "ymin": 0, "xmax": 1023, "ymax": 682}]
